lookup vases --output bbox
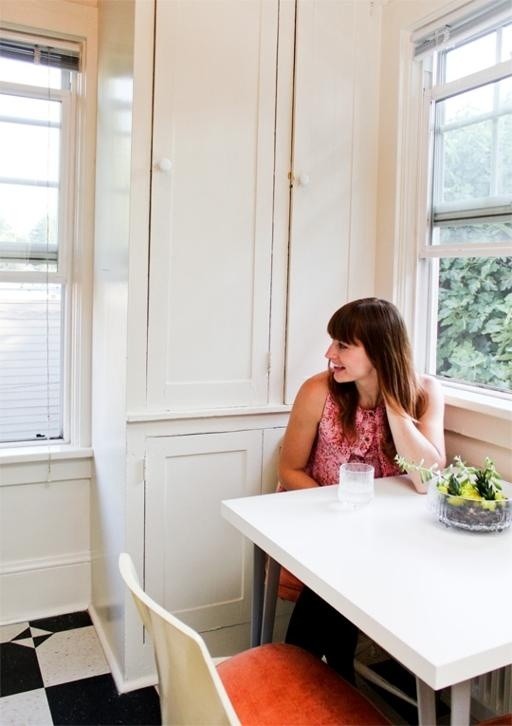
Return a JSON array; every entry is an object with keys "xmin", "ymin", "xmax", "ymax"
[{"xmin": 436, "ymin": 492, "xmax": 512, "ymax": 533}]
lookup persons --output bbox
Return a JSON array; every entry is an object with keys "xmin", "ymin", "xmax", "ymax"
[{"xmin": 278, "ymin": 298, "xmax": 446, "ymax": 681}]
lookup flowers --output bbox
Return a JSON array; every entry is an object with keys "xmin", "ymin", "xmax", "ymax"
[{"xmin": 392, "ymin": 454, "xmax": 506, "ymax": 512}]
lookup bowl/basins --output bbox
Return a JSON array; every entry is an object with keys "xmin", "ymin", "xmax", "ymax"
[{"xmin": 424, "ymin": 476, "xmax": 511, "ymax": 531}]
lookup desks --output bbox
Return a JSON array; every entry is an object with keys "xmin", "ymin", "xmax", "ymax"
[{"xmin": 221, "ymin": 468, "xmax": 512, "ymax": 726}]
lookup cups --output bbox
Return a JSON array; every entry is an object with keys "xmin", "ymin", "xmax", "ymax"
[{"xmin": 337, "ymin": 461, "xmax": 376, "ymax": 508}]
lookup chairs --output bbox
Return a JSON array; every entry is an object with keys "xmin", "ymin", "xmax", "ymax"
[
  {"xmin": 117, "ymin": 552, "xmax": 393, "ymax": 726},
  {"xmin": 261, "ymin": 482, "xmax": 305, "ymax": 644}
]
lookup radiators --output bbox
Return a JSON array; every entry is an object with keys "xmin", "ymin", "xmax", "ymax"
[{"xmin": 471, "ymin": 664, "xmax": 512, "ymax": 717}]
